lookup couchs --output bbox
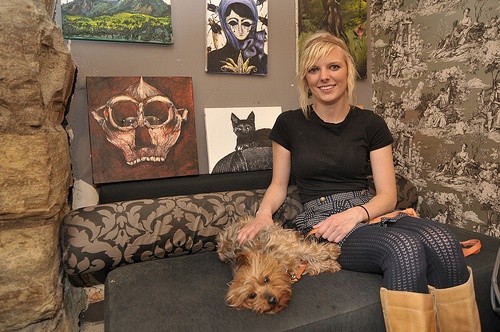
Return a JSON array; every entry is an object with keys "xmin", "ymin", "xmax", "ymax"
[{"xmin": 60, "ymin": 169, "xmax": 500, "ymax": 332}]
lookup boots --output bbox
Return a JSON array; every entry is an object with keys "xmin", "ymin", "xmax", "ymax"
[
  {"xmin": 428, "ymin": 265, "xmax": 482, "ymax": 332},
  {"xmin": 380, "ymin": 287, "xmax": 437, "ymax": 332}
]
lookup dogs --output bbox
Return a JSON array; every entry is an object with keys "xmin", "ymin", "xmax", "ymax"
[{"xmin": 214, "ymin": 214, "xmax": 343, "ymax": 315}]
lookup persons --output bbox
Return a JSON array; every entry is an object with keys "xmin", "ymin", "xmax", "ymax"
[{"xmin": 236, "ymin": 32, "xmax": 482, "ymax": 332}]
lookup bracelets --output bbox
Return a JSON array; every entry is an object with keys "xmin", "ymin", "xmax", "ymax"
[{"xmin": 357, "ymin": 205, "xmax": 371, "ymax": 222}]
